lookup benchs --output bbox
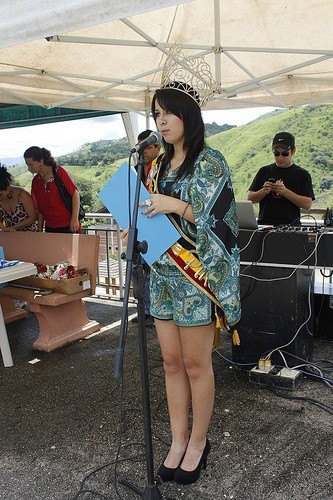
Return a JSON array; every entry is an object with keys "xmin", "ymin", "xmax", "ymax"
[{"xmin": 0, "ymin": 231, "xmax": 101, "ymax": 352}]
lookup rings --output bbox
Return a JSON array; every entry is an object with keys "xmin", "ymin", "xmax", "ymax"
[{"xmin": 144, "ymin": 199, "xmax": 152, "ymax": 206}]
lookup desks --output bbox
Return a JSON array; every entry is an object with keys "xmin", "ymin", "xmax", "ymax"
[{"xmin": 0, "ymin": 262, "xmax": 37, "ymax": 368}]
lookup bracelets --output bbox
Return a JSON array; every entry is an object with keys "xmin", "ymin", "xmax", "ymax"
[{"xmin": 181, "ymin": 202, "xmax": 190, "ymax": 218}]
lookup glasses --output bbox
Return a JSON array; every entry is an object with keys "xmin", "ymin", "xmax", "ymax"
[{"xmin": 273, "ymin": 147, "xmax": 291, "ymax": 156}]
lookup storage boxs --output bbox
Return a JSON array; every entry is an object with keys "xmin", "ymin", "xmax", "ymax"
[{"xmin": 12, "ymin": 272, "xmax": 90, "ymax": 294}]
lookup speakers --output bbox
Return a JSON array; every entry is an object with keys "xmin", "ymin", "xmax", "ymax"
[{"xmin": 233, "ymin": 266, "xmax": 314, "ymax": 375}]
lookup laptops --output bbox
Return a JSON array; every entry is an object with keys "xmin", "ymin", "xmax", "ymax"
[{"xmin": 235, "ymin": 201, "xmax": 274, "ymax": 230}]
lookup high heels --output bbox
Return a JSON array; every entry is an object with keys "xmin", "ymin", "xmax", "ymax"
[
  {"xmin": 173, "ymin": 438, "xmax": 211, "ymax": 484},
  {"xmin": 157, "ymin": 435, "xmax": 190, "ymax": 481}
]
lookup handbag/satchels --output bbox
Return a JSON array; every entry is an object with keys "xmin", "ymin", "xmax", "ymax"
[{"xmin": 52, "ymin": 165, "xmax": 85, "ymax": 219}]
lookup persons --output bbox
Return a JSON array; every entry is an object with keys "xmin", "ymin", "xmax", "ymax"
[
  {"xmin": 120, "ymin": 81, "xmax": 240, "ymax": 484},
  {"xmin": 0, "ymin": 130, "xmax": 163, "ymax": 327},
  {"xmin": 248, "ymin": 132, "xmax": 315, "ymax": 225}
]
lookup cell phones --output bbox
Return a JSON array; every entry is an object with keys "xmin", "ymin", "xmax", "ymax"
[{"xmin": 268, "ymin": 178, "xmax": 276, "ymax": 183}]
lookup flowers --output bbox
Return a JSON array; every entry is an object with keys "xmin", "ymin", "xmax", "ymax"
[{"xmin": 29, "ymin": 260, "xmax": 91, "ymax": 281}]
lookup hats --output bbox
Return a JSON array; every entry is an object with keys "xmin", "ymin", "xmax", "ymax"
[
  {"xmin": 135, "ymin": 130, "xmax": 160, "ymax": 146},
  {"xmin": 272, "ymin": 132, "xmax": 294, "ymax": 150}
]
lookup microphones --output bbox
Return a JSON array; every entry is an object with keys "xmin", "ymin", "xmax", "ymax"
[{"xmin": 130, "ymin": 131, "xmax": 162, "ymax": 152}]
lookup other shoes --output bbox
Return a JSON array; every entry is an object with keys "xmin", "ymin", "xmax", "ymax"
[
  {"xmin": 145, "ymin": 316, "xmax": 155, "ymax": 326},
  {"xmin": 131, "ymin": 315, "xmax": 150, "ymax": 322}
]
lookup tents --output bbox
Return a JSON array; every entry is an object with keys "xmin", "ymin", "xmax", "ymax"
[{"xmin": 0, "ymin": 0, "xmax": 333, "ymax": 165}]
